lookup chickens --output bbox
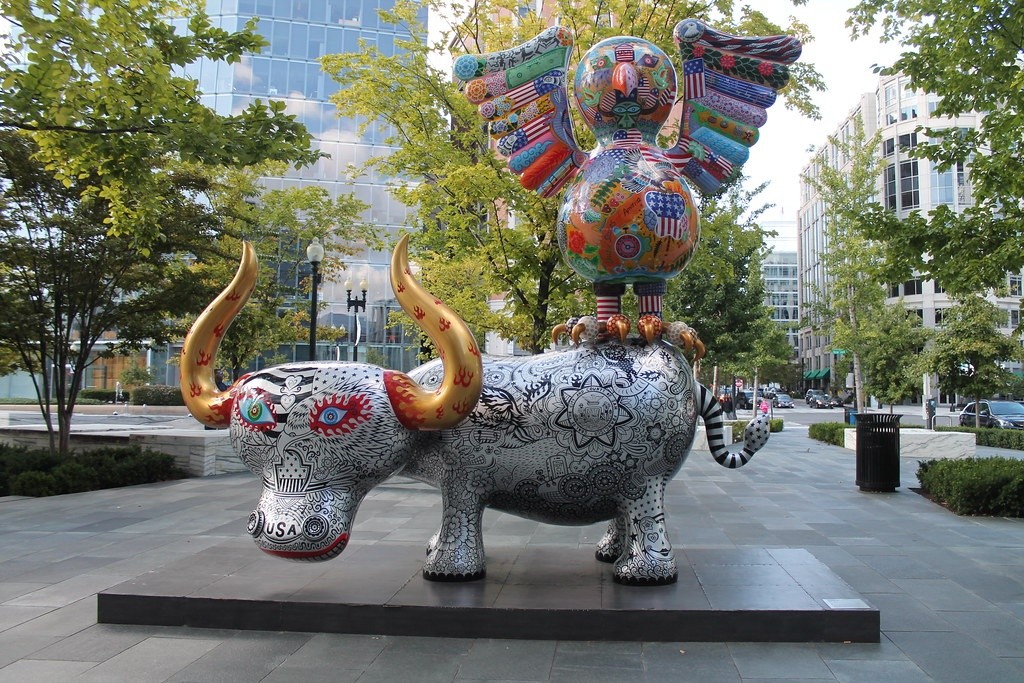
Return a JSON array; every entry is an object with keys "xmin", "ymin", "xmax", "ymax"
[{"xmin": 453, "ymin": 17, "xmax": 803, "ymax": 363}]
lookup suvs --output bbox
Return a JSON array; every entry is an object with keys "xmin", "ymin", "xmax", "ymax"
[
  {"xmin": 959, "ymin": 400, "xmax": 1024, "ymax": 430},
  {"xmin": 719, "ymin": 383, "xmax": 845, "ymax": 411}
]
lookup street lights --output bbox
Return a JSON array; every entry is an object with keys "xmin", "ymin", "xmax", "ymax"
[
  {"xmin": 306, "ymin": 235, "xmax": 325, "ymax": 360},
  {"xmin": 345, "ymin": 277, "xmax": 369, "ymax": 360}
]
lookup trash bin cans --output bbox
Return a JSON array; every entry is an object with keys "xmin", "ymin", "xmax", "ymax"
[{"xmin": 853, "ymin": 412, "xmax": 903, "ymax": 492}]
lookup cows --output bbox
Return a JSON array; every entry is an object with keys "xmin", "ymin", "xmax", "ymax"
[{"xmin": 180, "ymin": 237, "xmax": 771, "ymax": 587}]
